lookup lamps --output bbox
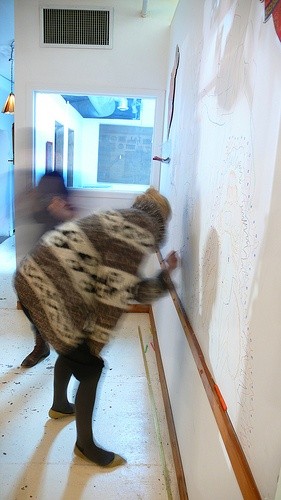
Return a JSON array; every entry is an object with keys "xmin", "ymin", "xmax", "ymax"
[{"xmin": 0, "ymin": 41, "xmax": 17, "ymax": 115}]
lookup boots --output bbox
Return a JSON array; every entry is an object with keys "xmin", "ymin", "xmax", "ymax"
[{"xmin": 21, "ymin": 333, "xmax": 50, "ymax": 369}]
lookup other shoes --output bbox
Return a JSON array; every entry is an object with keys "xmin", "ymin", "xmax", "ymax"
[
  {"xmin": 48, "ymin": 403, "xmax": 75, "ymax": 419},
  {"xmin": 73, "ymin": 443, "xmax": 127, "ymax": 468}
]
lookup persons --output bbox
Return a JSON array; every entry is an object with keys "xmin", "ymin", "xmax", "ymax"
[
  {"xmin": 14, "ymin": 188, "xmax": 179, "ymax": 467},
  {"xmin": 18, "ymin": 169, "xmax": 79, "ymax": 368}
]
